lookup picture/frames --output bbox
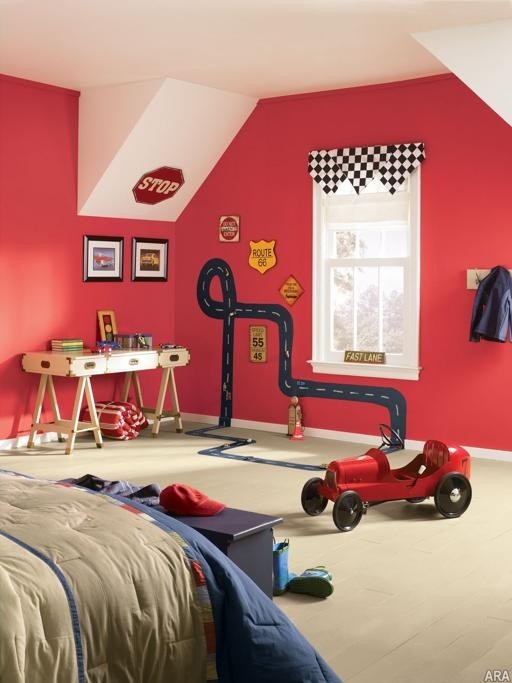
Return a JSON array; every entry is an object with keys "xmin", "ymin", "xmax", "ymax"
[
  {"xmin": 81, "ymin": 230, "xmax": 125, "ymax": 283},
  {"xmin": 129, "ymin": 235, "xmax": 171, "ymax": 282}
]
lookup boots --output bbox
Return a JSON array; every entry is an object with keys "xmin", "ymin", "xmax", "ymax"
[
  {"xmin": 288, "ymin": 566, "xmax": 334, "ymax": 599},
  {"xmin": 273, "ymin": 538, "xmax": 297, "ymax": 596}
]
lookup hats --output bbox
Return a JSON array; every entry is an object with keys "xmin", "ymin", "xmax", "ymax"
[{"xmin": 160, "ymin": 483, "xmax": 225, "ymax": 517}]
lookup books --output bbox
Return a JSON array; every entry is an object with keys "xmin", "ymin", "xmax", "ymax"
[{"xmin": 51, "ymin": 338, "xmax": 84, "ymax": 351}]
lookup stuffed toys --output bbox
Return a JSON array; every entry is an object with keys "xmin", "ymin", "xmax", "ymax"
[
  {"xmin": 137, "ymin": 336, "xmax": 149, "ymax": 348},
  {"xmin": 301, "ymin": 423, "xmax": 473, "ymax": 531},
  {"xmin": 91, "ymin": 340, "xmax": 121, "ymax": 353},
  {"xmin": 161, "ymin": 343, "xmax": 177, "ymax": 348}
]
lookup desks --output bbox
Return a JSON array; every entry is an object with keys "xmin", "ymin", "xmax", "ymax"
[
  {"xmin": 137, "ymin": 491, "xmax": 285, "ymax": 611},
  {"xmin": 19, "ymin": 339, "xmax": 191, "ymax": 455}
]
